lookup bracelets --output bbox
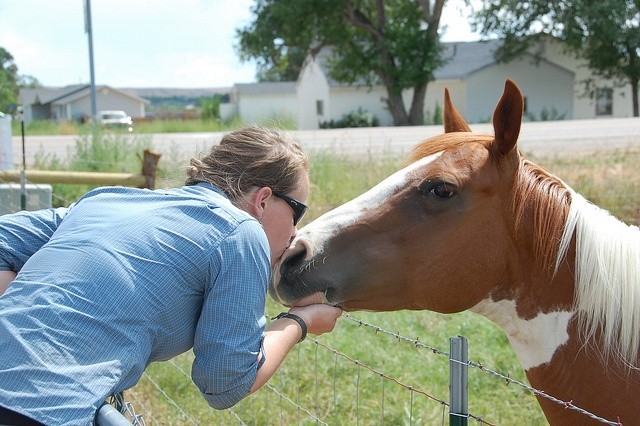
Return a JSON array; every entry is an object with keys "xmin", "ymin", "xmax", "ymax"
[{"xmin": 272, "ymin": 312, "xmax": 307, "ymax": 342}]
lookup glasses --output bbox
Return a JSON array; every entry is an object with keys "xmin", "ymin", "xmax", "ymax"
[{"xmin": 259, "ymin": 185, "xmax": 308, "ymax": 226}]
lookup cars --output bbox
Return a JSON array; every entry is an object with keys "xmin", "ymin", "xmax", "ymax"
[{"xmin": 101, "ymin": 111, "xmax": 132, "ymax": 125}]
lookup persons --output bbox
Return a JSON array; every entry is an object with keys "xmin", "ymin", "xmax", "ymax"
[{"xmin": 1, "ymin": 128, "xmax": 343, "ymax": 425}]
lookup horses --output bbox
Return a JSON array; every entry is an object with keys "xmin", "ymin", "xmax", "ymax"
[{"xmin": 266, "ymin": 76, "xmax": 639, "ymax": 426}]
